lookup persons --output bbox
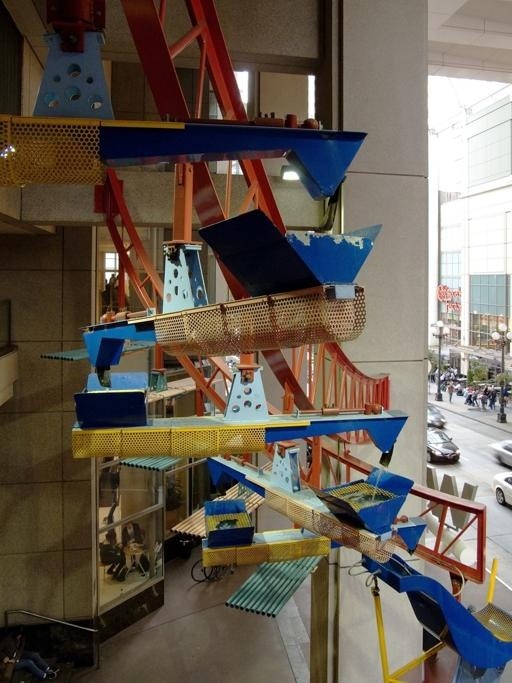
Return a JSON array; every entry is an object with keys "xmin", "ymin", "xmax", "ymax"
[
  {"xmin": 121, "ymin": 519, "xmax": 148, "ymax": 577},
  {"xmin": 108, "ymin": 273, "xmax": 117, "ymax": 286},
  {"xmin": 114, "ymin": 274, "xmax": 119, "ymax": 288},
  {"xmin": 1, "ymin": 630, "xmax": 59, "ymax": 682},
  {"xmin": 429, "ymin": 365, "xmax": 510, "ymax": 411}
]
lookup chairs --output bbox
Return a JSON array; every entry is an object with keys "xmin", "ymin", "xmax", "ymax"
[
  {"xmin": 138, "ymin": 540, "xmax": 162, "ymax": 573},
  {"xmin": 100, "ymin": 543, "xmax": 125, "ymax": 574}
]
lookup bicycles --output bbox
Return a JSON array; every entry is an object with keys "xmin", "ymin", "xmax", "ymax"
[
  {"xmin": 189, "ymin": 549, "xmax": 240, "ymax": 582},
  {"xmin": 167, "ymin": 479, "xmax": 184, "ymax": 507}
]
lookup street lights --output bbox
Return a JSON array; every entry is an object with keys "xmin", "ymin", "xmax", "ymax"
[
  {"xmin": 430, "ymin": 319, "xmax": 451, "ymax": 400},
  {"xmin": 491, "ymin": 322, "xmax": 512, "ymax": 424}
]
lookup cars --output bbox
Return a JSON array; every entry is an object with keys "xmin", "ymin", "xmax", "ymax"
[
  {"xmin": 427, "ymin": 402, "xmax": 448, "ymax": 429},
  {"xmin": 492, "ymin": 471, "xmax": 511, "ymax": 507},
  {"xmin": 428, "ymin": 426, "xmax": 462, "ymax": 465},
  {"xmin": 487, "ymin": 439, "xmax": 511, "ymax": 466}
]
